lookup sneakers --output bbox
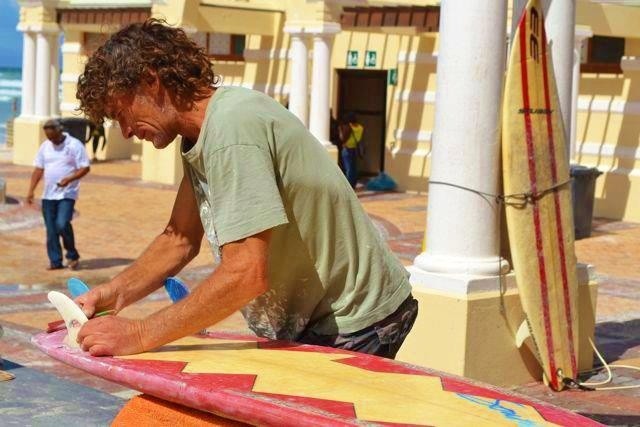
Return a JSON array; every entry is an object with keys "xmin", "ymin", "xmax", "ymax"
[{"xmin": 69, "ymin": 260, "xmax": 80, "ymax": 270}]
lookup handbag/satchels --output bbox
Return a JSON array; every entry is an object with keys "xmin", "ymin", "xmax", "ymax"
[{"xmin": 350, "ymin": 126, "xmax": 366, "ymax": 159}]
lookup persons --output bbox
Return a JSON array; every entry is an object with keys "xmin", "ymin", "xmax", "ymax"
[
  {"xmin": 337, "ymin": 112, "xmax": 363, "ymax": 191},
  {"xmin": 25, "ymin": 119, "xmax": 90, "ymax": 271},
  {"xmin": 71, "ymin": 16, "xmax": 419, "ymax": 361}
]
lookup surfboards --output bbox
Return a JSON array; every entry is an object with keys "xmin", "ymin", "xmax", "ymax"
[
  {"xmin": 501, "ymin": 3, "xmax": 579, "ymax": 392},
  {"xmin": 30, "ymin": 314, "xmax": 612, "ymax": 427}
]
